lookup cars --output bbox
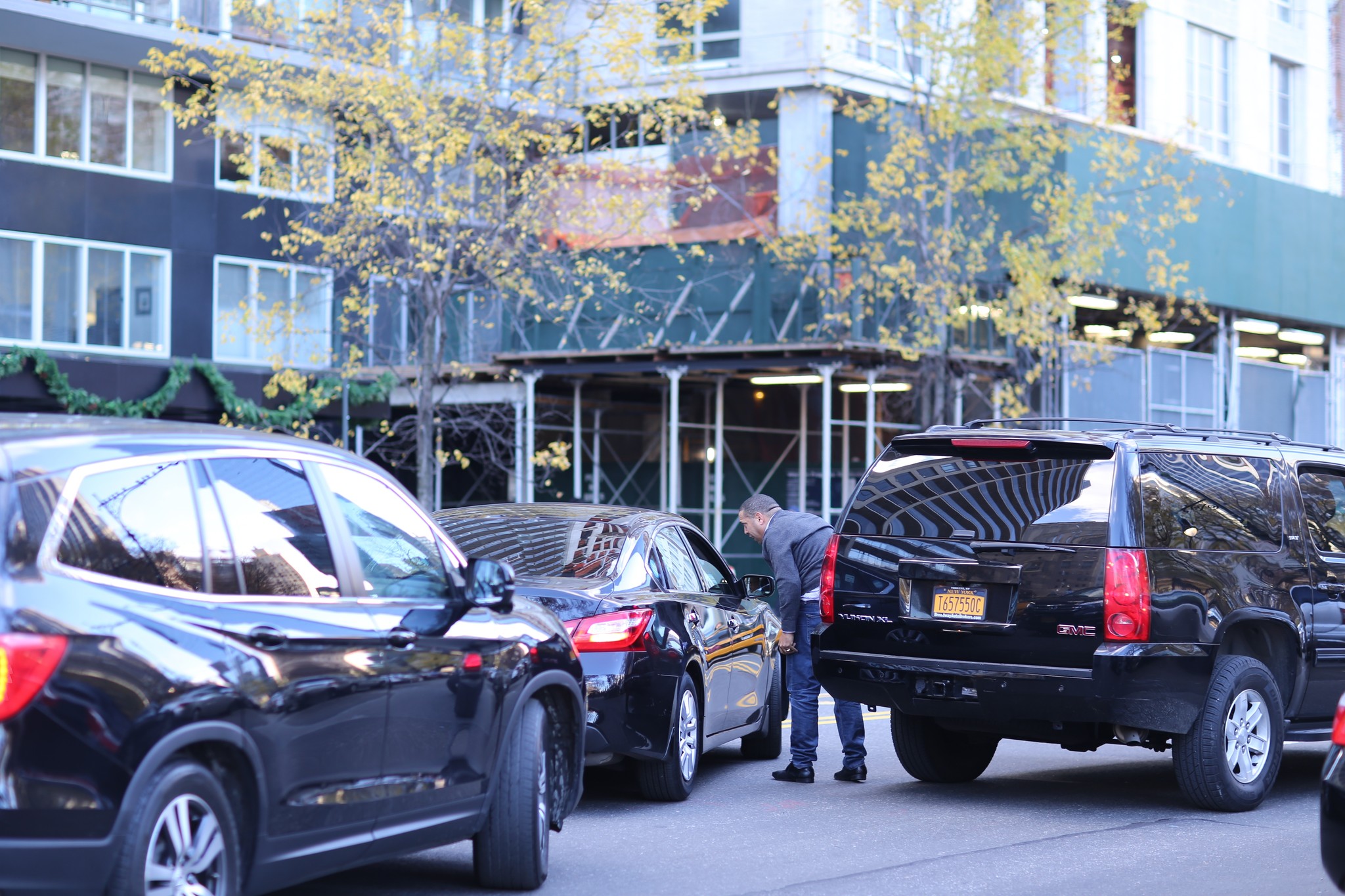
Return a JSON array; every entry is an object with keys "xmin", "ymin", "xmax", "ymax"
[{"xmin": 431, "ymin": 501, "xmax": 787, "ymax": 802}]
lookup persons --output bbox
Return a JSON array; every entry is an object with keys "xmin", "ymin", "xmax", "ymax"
[{"xmin": 737, "ymin": 493, "xmax": 868, "ymax": 783}]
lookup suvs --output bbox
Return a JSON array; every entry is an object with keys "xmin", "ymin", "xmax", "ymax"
[
  {"xmin": 809, "ymin": 416, "xmax": 1344, "ymax": 811},
  {"xmin": 2, "ymin": 409, "xmax": 588, "ymax": 896}
]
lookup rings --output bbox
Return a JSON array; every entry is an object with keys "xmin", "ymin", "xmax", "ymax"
[{"xmin": 786, "ymin": 648, "xmax": 790, "ymax": 652}]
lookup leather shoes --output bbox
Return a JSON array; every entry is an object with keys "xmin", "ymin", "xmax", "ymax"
[
  {"xmin": 771, "ymin": 761, "xmax": 816, "ymax": 782},
  {"xmin": 834, "ymin": 766, "xmax": 870, "ymax": 780}
]
